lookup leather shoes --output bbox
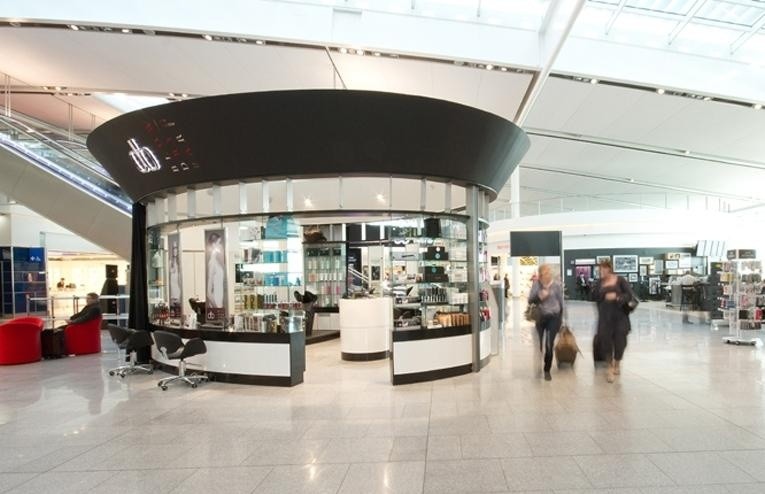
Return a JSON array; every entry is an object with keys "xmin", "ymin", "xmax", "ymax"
[{"xmin": 545, "ymin": 371, "xmax": 551, "ymax": 381}]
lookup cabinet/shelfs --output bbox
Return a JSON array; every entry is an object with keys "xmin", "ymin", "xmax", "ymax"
[
  {"xmin": 716, "ymin": 243, "xmax": 765, "ymax": 347},
  {"xmin": 339, "ymin": 295, "xmax": 396, "ymax": 362},
  {"xmin": 390, "ymin": 210, "xmax": 471, "ymax": 333},
  {"xmin": 302, "ymin": 241, "xmax": 350, "ymax": 333},
  {"xmin": 477, "ymin": 215, "xmax": 494, "ymax": 324},
  {"xmin": 235, "ymin": 219, "xmax": 302, "ymax": 336},
  {"xmin": 0, "ymin": 244, "xmax": 48, "ymax": 318}
]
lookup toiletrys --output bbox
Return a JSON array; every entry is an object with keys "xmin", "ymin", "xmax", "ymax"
[{"xmin": 147, "ymin": 225, "xmax": 490, "ymax": 332}]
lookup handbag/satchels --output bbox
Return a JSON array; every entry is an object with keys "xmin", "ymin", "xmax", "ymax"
[
  {"xmin": 524, "ymin": 302, "xmax": 542, "ymax": 321},
  {"xmin": 623, "ymin": 296, "xmax": 638, "ymax": 311}
]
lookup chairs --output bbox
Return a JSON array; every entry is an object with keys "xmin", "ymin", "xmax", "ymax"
[
  {"xmin": 0, "ymin": 315, "xmax": 45, "ymax": 367},
  {"xmin": 679, "ymin": 283, "xmax": 697, "ymax": 313},
  {"xmin": 105, "ymin": 320, "xmax": 155, "ymax": 378},
  {"xmin": 153, "ymin": 329, "xmax": 208, "ymax": 391},
  {"xmin": 65, "ymin": 310, "xmax": 102, "ymax": 357}
]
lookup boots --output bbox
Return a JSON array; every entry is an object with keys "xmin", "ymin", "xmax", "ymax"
[
  {"xmin": 606, "ymin": 365, "xmax": 615, "ymax": 383},
  {"xmin": 613, "ymin": 360, "xmax": 620, "ymax": 375}
]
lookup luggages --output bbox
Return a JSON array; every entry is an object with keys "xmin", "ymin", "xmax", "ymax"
[
  {"xmin": 555, "ymin": 329, "xmax": 577, "ymax": 367},
  {"xmin": 594, "ymin": 335, "xmax": 606, "ymax": 361}
]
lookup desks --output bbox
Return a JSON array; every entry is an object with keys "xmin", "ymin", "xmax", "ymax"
[{"xmin": 40, "ymin": 327, "xmax": 65, "ymax": 362}]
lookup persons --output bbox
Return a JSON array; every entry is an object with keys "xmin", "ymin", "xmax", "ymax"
[
  {"xmin": 527, "ymin": 263, "xmax": 564, "ymax": 381},
  {"xmin": 576, "ymin": 272, "xmax": 592, "ymax": 301},
  {"xmin": 57, "ymin": 293, "xmax": 101, "ymax": 356},
  {"xmin": 596, "ymin": 263, "xmax": 634, "ymax": 381}
]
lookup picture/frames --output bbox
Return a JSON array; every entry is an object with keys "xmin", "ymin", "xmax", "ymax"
[{"xmin": 595, "ymin": 252, "xmax": 679, "ymax": 295}]
